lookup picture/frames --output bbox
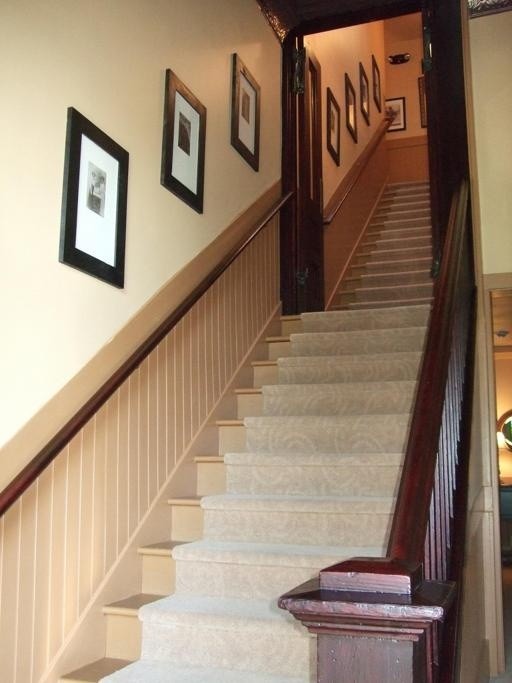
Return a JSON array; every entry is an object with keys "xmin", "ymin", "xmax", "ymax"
[
  {"xmin": 325, "ymin": 52, "xmax": 382, "ymax": 166},
  {"xmin": 56, "ymin": 104, "xmax": 132, "ymax": 291},
  {"xmin": 229, "ymin": 48, "xmax": 263, "ymax": 171},
  {"xmin": 158, "ymin": 66, "xmax": 209, "ymax": 216},
  {"xmin": 384, "ymin": 95, "xmax": 407, "ymax": 132},
  {"xmin": 417, "ymin": 75, "xmax": 428, "ymax": 129}
]
